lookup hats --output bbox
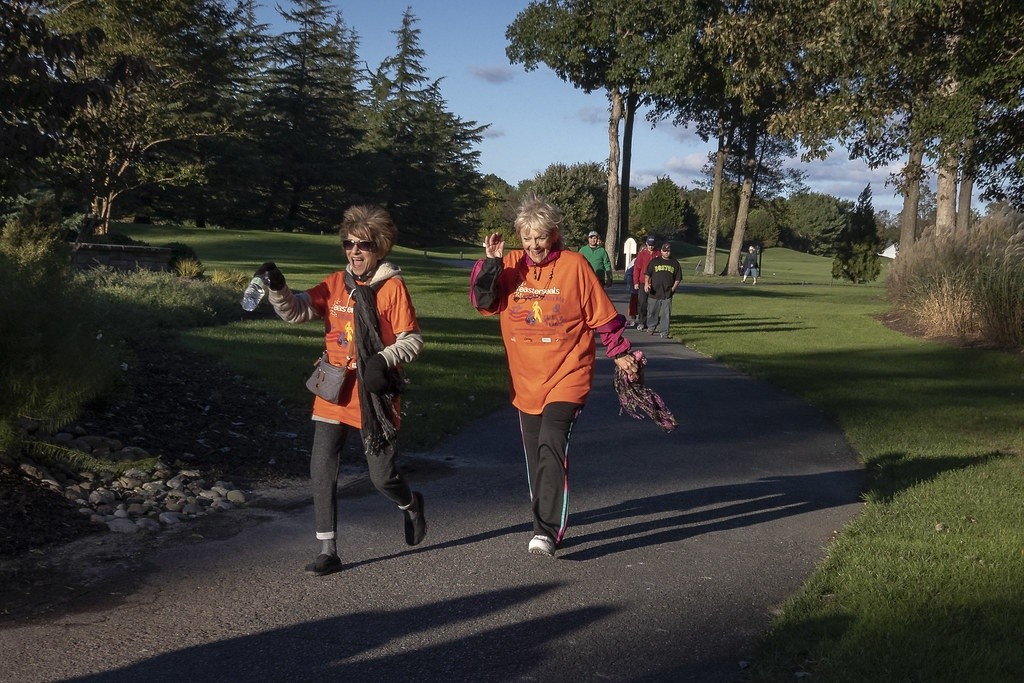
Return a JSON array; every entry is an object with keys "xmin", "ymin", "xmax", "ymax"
[
  {"xmin": 588, "ymin": 231, "xmax": 598, "ymax": 237},
  {"xmin": 647, "ymin": 236, "xmax": 656, "ymax": 245},
  {"xmin": 662, "ymin": 244, "xmax": 671, "ymax": 252}
]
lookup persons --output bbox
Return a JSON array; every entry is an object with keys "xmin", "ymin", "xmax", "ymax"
[
  {"xmin": 632, "ymin": 236, "xmax": 662, "ymax": 331},
  {"xmin": 577, "ymin": 230, "xmax": 612, "ymax": 292},
  {"xmin": 623, "ymin": 256, "xmax": 640, "ymax": 326},
  {"xmin": 595, "ymin": 235, "xmax": 603, "ymax": 246},
  {"xmin": 253, "ymin": 203, "xmax": 428, "ymax": 576},
  {"xmin": 468, "ymin": 195, "xmax": 640, "ymax": 557},
  {"xmin": 644, "ymin": 242, "xmax": 684, "ymax": 339},
  {"xmin": 741, "ymin": 246, "xmax": 758, "ymax": 285}
]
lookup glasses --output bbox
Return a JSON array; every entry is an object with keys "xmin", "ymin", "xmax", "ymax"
[{"xmin": 343, "ymin": 239, "xmax": 376, "ymax": 251}]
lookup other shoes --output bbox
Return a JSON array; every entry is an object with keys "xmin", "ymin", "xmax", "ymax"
[
  {"xmin": 305, "ymin": 553, "xmax": 342, "ymax": 574},
  {"xmin": 636, "ymin": 324, "xmax": 645, "ymax": 331},
  {"xmin": 404, "ymin": 491, "xmax": 426, "ymax": 546},
  {"xmin": 741, "ymin": 280, "xmax": 757, "ymax": 286},
  {"xmin": 660, "ymin": 332, "xmax": 668, "ymax": 338},
  {"xmin": 647, "ymin": 328, "xmax": 653, "ymax": 335},
  {"xmin": 528, "ymin": 535, "xmax": 557, "ymax": 556},
  {"xmin": 630, "ymin": 320, "xmax": 638, "ymax": 327}
]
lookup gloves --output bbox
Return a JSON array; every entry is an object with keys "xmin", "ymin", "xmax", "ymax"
[
  {"xmin": 254, "ymin": 261, "xmax": 284, "ymax": 291},
  {"xmin": 364, "ymin": 355, "xmax": 389, "ymax": 395}
]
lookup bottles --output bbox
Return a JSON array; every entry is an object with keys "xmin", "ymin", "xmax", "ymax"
[{"xmin": 241, "ymin": 270, "xmax": 271, "ymax": 312}]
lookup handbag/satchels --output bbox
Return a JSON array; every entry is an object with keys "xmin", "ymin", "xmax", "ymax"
[{"xmin": 306, "ymin": 353, "xmax": 347, "ymax": 404}]
